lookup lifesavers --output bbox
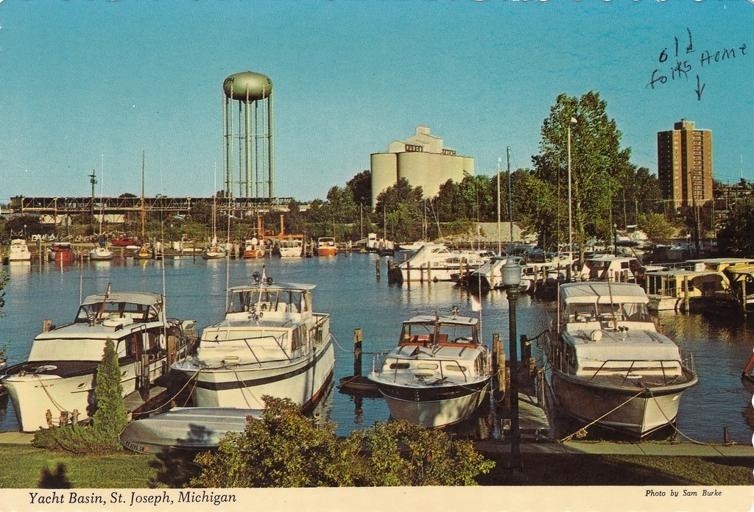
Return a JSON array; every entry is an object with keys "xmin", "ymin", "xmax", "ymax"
[
  {"xmin": 160, "ymin": 333, "xmax": 167, "ymax": 350},
  {"xmin": 103, "ymin": 318, "xmax": 133, "ymax": 327}
]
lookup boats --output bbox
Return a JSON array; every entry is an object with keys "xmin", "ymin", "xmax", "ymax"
[
  {"xmin": 0, "ymin": 284, "xmax": 199, "ymax": 431},
  {"xmin": 168, "ymin": 263, "xmax": 338, "ymax": 418},
  {"xmin": 367, "ymin": 310, "xmax": 497, "ymax": 434},
  {"xmin": 542, "ymin": 281, "xmax": 700, "ymax": 440}
]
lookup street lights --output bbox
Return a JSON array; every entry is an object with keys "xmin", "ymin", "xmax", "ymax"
[
  {"xmin": 566, "ymin": 116, "xmax": 578, "ymax": 252},
  {"xmin": 496, "ymin": 159, "xmax": 501, "ymax": 255},
  {"xmin": 501, "ymin": 256, "xmax": 524, "ymax": 469}
]
